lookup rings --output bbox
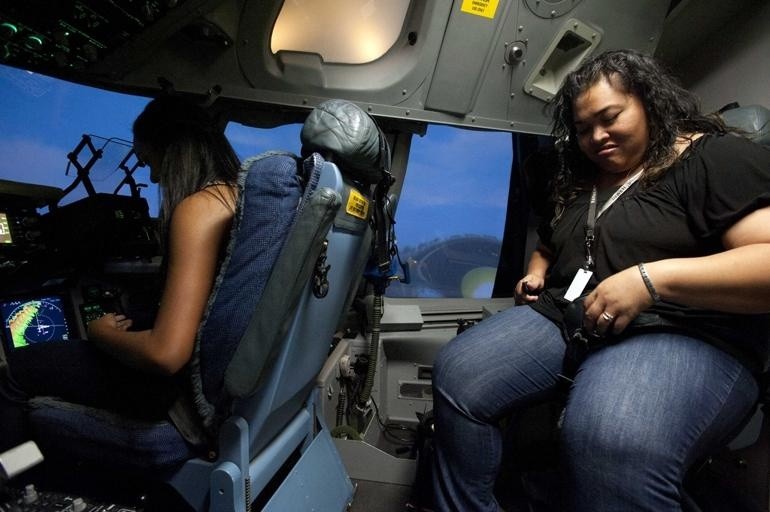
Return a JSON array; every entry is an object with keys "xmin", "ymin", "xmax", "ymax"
[{"xmin": 602, "ymin": 311, "xmax": 614, "ymax": 323}]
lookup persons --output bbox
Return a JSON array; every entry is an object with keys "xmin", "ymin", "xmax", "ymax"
[
  {"xmin": 0, "ymin": 89, "xmax": 243, "ymax": 491},
  {"xmin": 417, "ymin": 47, "xmax": 768, "ymax": 511}
]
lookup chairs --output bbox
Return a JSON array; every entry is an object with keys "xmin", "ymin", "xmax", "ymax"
[
  {"xmin": 687, "ymin": 100, "xmax": 768, "ymax": 511},
  {"xmin": 18, "ymin": 98, "xmax": 394, "ymax": 512}
]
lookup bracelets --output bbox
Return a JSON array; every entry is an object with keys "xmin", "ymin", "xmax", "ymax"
[{"xmin": 636, "ymin": 261, "xmax": 663, "ymax": 307}]
